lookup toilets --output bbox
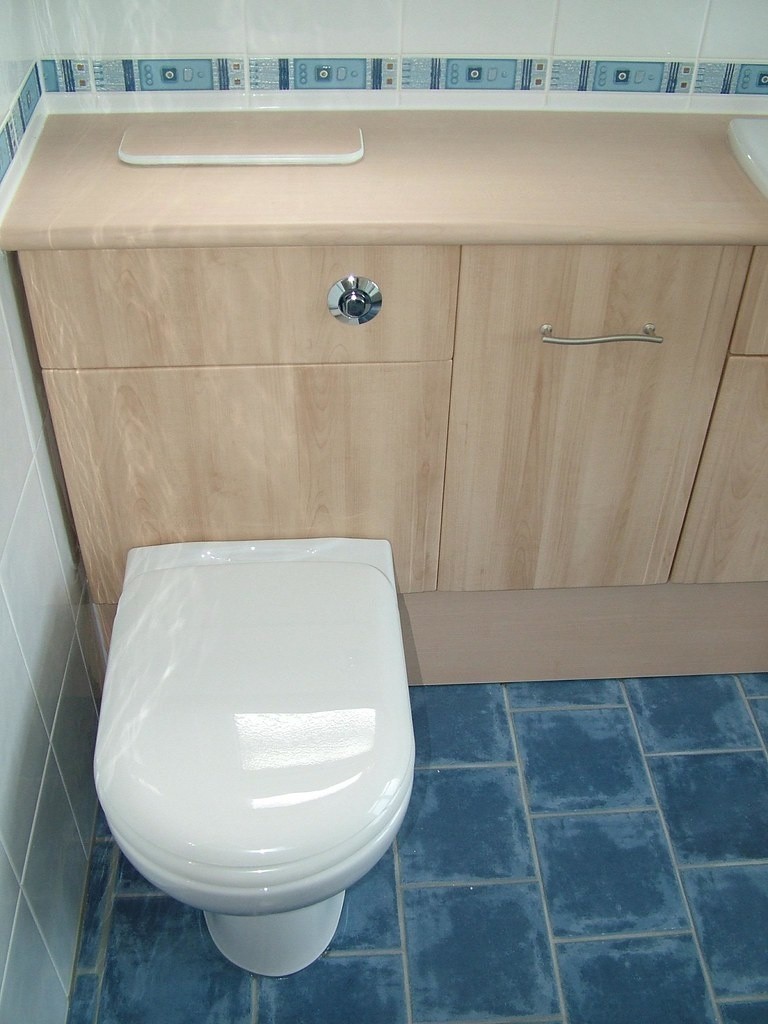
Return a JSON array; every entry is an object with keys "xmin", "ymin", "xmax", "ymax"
[{"xmin": 87, "ymin": 536, "xmax": 424, "ymax": 983}]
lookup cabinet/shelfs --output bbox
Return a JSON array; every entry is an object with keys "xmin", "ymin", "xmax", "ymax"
[{"xmin": 13, "ymin": 243, "xmax": 768, "ymax": 687}]
